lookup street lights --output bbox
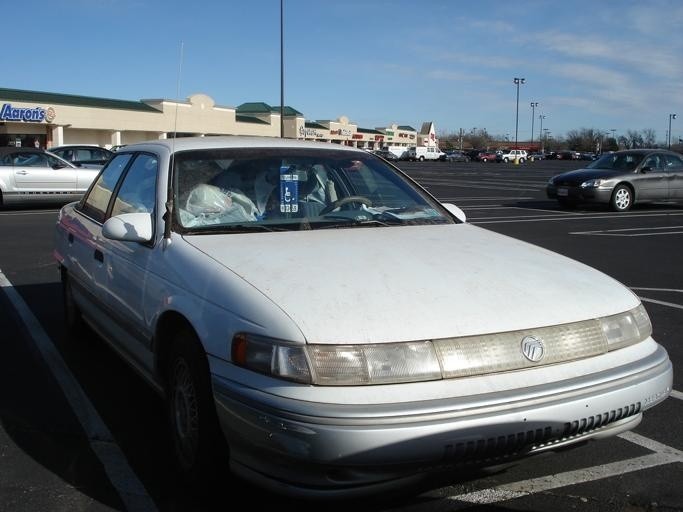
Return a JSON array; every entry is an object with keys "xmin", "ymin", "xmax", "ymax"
[
  {"xmin": 611, "ymin": 128, "xmax": 616, "ymax": 139},
  {"xmin": 514, "ymin": 78, "xmax": 525, "ymax": 148},
  {"xmin": 668, "ymin": 112, "xmax": 676, "ymax": 148},
  {"xmin": 538, "ymin": 116, "xmax": 546, "ymax": 140},
  {"xmin": 530, "ymin": 102, "xmax": 538, "ymax": 143},
  {"xmin": 543, "ymin": 128, "xmax": 553, "ymax": 141}
]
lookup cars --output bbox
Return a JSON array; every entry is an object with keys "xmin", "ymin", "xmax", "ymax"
[
  {"xmin": 19, "ymin": 146, "xmax": 113, "ymax": 168},
  {"xmin": 51, "ymin": 134, "xmax": 675, "ymax": 504},
  {"xmin": 361, "ymin": 146, "xmax": 599, "ymax": 165},
  {"xmin": 0, "ymin": 145, "xmax": 105, "ymax": 209},
  {"xmin": 545, "ymin": 148, "xmax": 683, "ymax": 212}
]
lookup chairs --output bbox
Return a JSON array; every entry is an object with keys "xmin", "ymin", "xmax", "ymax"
[{"xmin": 265, "ymin": 164, "xmax": 334, "ymax": 221}]
